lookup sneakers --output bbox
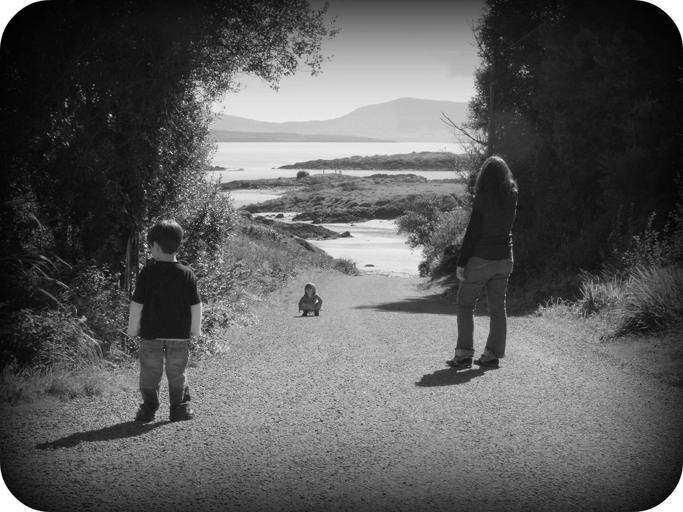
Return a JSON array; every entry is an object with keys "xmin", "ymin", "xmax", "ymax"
[
  {"xmin": 476, "ymin": 355, "xmax": 500, "ymax": 367},
  {"xmin": 445, "ymin": 358, "xmax": 471, "ymax": 366},
  {"xmin": 170, "ymin": 409, "xmax": 192, "ymax": 421},
  {"xmin": 137, "ymin": 409, "xmax": 156, "ymax": 420}
]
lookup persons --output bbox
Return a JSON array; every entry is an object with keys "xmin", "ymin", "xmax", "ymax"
[
  {"xmin": 126, "ymin": 218, "xmax": 203, "ymax": 424},
  {"xmin": 298, "ymin": 282, "xmax": 321, "ymax": 318},
  {"xmin": 445, "ymin": 155, "xmax": 518, "ymax": 370}
]
